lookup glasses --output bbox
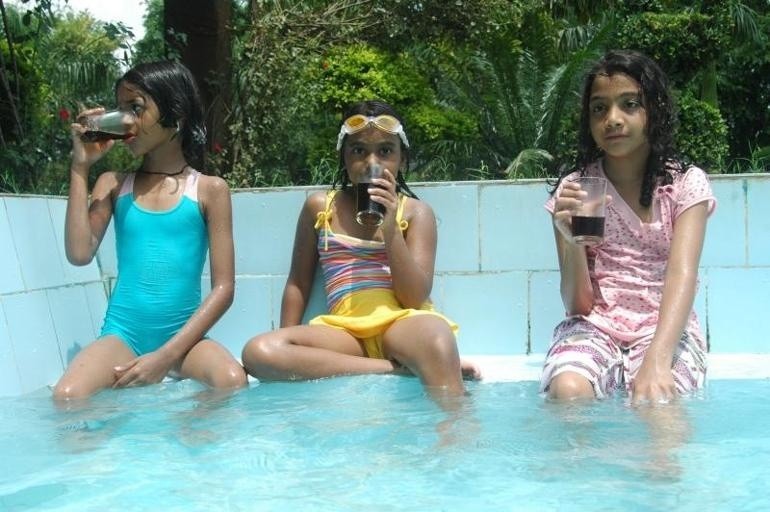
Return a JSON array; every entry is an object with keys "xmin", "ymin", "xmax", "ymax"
[{"xmin": 335, "ymin": 114, "xmax": 410, "ymax": 152}]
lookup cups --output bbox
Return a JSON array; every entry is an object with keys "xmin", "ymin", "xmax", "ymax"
[
  {"xmin": 570, "ymin": 176, "xmax": 608, "ymax": 249},
  {"xmin": 78, "ymin": 105, "xmax": 139, "ymax": 144},
  {"xmin": 353, "ymin": 162, "xmax": 393, "ymax": 228}
]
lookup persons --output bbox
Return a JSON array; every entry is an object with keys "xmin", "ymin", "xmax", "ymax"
[
  {"xmin": 240, "ymin": 99, "xmax": 482, "ymax": 407},
  {"xmin": 536, "ymin": 47, "xmax": 718, "ymax": 413},
  {"xmin": 51, "ymin": 58, "xmax": 249, "ymax": 405}
]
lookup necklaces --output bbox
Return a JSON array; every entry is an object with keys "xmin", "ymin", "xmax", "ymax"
[{"xmin": 140, "ymin": 164, "xmax": 190, "ymax": 177}]
